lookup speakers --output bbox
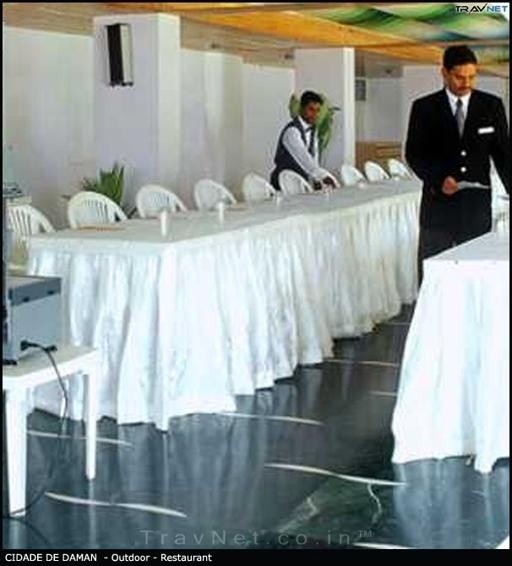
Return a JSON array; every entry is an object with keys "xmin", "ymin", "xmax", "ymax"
[{"xmin": 105, "ymin": 22, "xmax": 134, "ymax": 89}]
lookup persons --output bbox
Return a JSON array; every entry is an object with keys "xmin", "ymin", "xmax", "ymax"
[
  {"xmin": 271, "ymin": 90, "xmax": 340, "ymax": 191},
  {"xmin": 404, "ymin": 43, "xmax": 509, "ymax": 288}
]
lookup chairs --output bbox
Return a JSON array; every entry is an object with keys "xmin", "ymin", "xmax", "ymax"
[{"xmin": 7, "ymin": 159, "xmax": 408, "ymax": 274}]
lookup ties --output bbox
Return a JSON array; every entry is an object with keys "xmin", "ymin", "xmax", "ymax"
[{"xmin": 455, "ymin": 99, "xmax": 465, "ymax": 141}]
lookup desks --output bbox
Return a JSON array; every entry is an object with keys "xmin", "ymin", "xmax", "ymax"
[
  {"xmin": 2, "ymin": 345, "xmax": 96, "ymax": 519},
  {"xmin": 28, "ymin": 172, "xmax": 424, "ymax": 430},
  {"xmin": 391, "ymin": 231, "xmax": 510, "ymax": 474}
]
